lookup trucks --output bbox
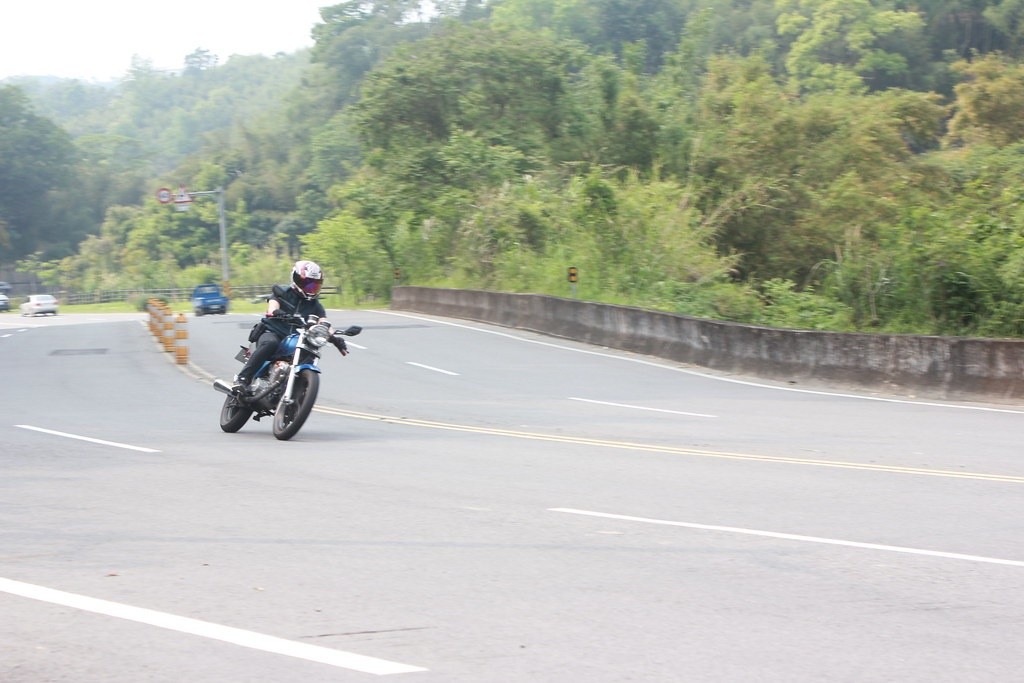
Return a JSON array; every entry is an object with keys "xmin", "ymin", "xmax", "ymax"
[{"xmin": 190, "ymin": 283, "xmax": 230, "ymax": 316}]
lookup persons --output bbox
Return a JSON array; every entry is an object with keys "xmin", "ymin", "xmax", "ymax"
[{"xmin": 231, "ymin": 259, "xmax": 349, "ymax": 397}]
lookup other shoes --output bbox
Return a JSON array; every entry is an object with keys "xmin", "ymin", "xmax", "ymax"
[{"xmin": 231, "ymin": 375, "xmax": 247, "ymax": 391}]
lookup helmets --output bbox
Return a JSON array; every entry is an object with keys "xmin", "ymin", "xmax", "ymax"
[{"xmin": 290, "ymin": 260, "xmax": 324, "ymax": 302}]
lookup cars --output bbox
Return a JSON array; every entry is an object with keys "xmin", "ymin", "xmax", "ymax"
[
  {"xmin": 0, "ymin": 293, "xmax": 10, "ymax": 312},
  {"xmin": 0, "ymin": 281, "xmax": 12, "ymax": 294},
  {"xmin": 19, "ymin": 294, "xmax": 59, "ymax": 319}
]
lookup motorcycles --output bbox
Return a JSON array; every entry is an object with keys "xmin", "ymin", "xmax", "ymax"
[{"xmin": 213, "ymin": 285, "xmax": 362, "ymax": 440}]
